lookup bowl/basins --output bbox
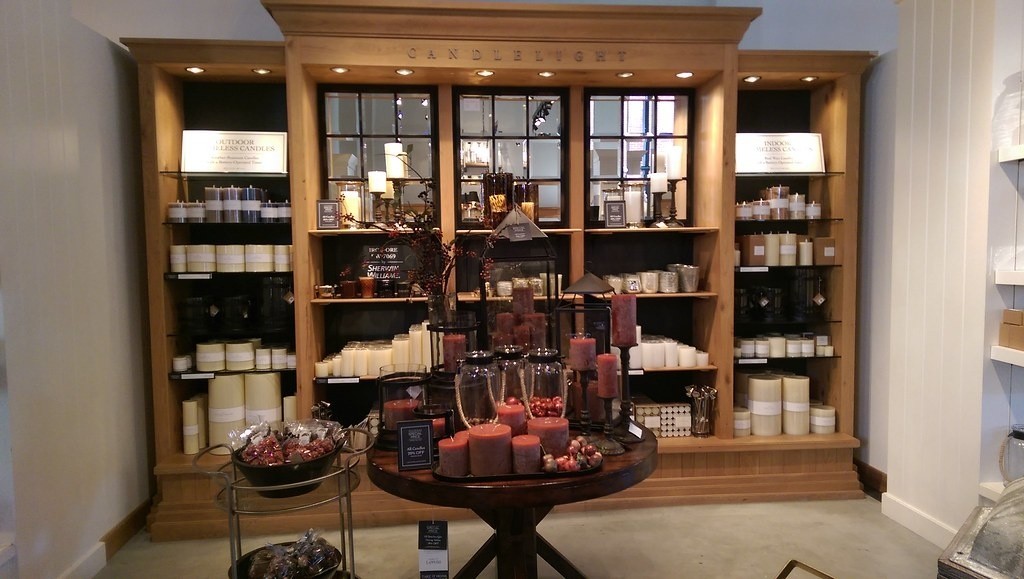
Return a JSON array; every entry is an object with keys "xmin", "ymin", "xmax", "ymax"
[{"xmin": 232, "ymin": 439, "xmax": 346, "ymax": 498}]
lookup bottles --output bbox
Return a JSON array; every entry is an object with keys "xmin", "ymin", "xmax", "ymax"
[
  {"xmin": 460, "ymin": 344, "xmax": 568, "ymax": 425},
  {"xmin": 1002, "ymin": 424, "xmax": 1024, "ymax": 486}
]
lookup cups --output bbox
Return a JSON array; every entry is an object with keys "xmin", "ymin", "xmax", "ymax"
[{"xmin": 692, "ymin": 398, "xmax": 712, "ymax": 437}]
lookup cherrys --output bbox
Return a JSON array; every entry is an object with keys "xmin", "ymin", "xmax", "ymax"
[
  {"xmin": 506, "ymin": 395, "xmax": 563, "ymax": 417},
  {"xmin": 541, "ymin": 435, "xmax": 603, "ymax": 473}
]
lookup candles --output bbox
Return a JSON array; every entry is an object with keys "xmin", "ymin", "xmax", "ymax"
[{"xmin": 169, "ymin": 143, "xmax": 836, "ymax": 480}]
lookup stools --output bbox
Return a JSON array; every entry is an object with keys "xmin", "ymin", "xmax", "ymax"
[{"xmin": 937, "ymin": 506, "xmax": 1024, "ymax": 579}]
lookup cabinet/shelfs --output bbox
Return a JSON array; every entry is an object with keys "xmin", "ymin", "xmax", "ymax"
[
  {"xmin": 115, "ymin": 0, "xmax": 866, "ymax": 544},
  {"xmin": 192, "ymin": 428, "xmax": 374, "ymax": 579},
  {"xmin": 978, "ymin": 143, "xmax": 1024, "ymax": 504}
]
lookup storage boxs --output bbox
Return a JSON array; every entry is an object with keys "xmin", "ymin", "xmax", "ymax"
[{"xmin": 998, "ymin": 309, "xmax": 1024, "ymax": 350}]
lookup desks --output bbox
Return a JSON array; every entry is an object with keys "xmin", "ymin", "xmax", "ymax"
[{"xmin": 367, "ymin": 409, "xmax": 660, "ymax": 579}]
